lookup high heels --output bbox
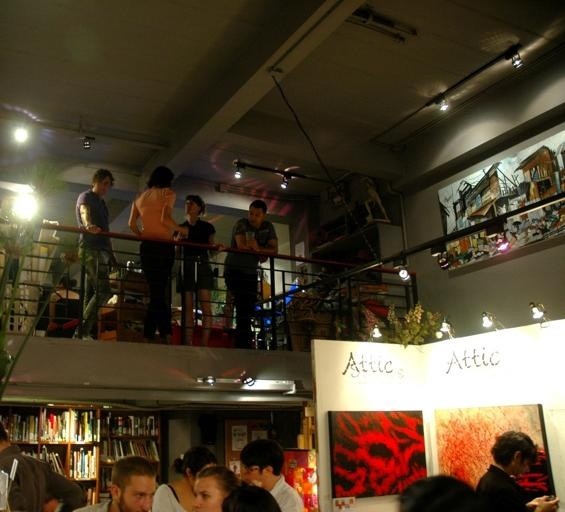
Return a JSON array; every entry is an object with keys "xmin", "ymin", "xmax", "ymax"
[
  {"xmin": 200, "ymin": 342, "xmax": 208, "ymax": 347},
  {"xmin": 186, "ymin": 340, "xmax": 193, "ymax": 346}
]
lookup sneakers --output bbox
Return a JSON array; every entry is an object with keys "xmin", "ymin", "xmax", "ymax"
[
  {"xmin": 81, "ymin": 333, "xmax": 95, "ymax": 341},
  {"xmin": 145, "ymin": 335, "xmax": 155, "ymax": 343},
  {"xmin": 160, "ymin": 335, "xmax": 171, "ymax": 345}
]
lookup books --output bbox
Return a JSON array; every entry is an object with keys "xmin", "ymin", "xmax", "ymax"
[
  {"xmin": 97, "ymin": 463, "xmax": 112, "ymax": 494},
  {"xmin": 100, "ymin": 414, "xmax": 164, "ymax": 439},
  {"xmin": 70, "ymin": 446, "xmax": 96, "ymax": 480},
  {"xmin": 39, "ymin": 445, "xmax": 66, "ymax": 479},
  {"xmin": 101, "ymin": 438, "xmax": 163, "ymax": 462},
  {"xmin": 43, "ymin": 410, "xmax": 97, "ymax": 444},
  {"xmin": 2, "ymin": 413, "xmax": 40, "ymax": 442}
]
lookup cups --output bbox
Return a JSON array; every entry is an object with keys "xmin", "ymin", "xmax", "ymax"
[{"xmin": 246, "ymin": 231, "xmax": 255, "ymax": 246}]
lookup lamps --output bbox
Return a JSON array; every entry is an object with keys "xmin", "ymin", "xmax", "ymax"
[
  {"xmin": 234, "ymin": 166, "xmax": 241, "ymax": 179},
  {"xmin": 528, "ymin": 300, "xmax": 551, "ymax": 323},
  {"xmin": 372, "ymin": 325, "xmax": 382, "ymax": 337},
  {"xmin": 437, "ymin": 255, "xmax": 450, "ymax": 269},
  {"xmin": 439, "ymin": 99, "xmax": 448, "ymax": 111},
  {"xmin": 430, "ymin": 244, "xmax": 441, "ymax": 257},
  {"xmin": 399, "ymin": 267, "xmax": 410, "ymax": 281},
  {"xmin": 280, "ymin": 180, "xmax": 288, "ymax": 189},
  {"xmin": 511, "ymin": 53, "xmax": 523, "ymax": 69},
  {"xmin": 439, "ymin": 315, "xmax": 451, "ymax": 332},
  {"xmin": 481, "ymin": 310, "xmax": 505, "ymax": 331}
]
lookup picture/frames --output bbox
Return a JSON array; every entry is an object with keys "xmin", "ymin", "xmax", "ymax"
[
  {"xmin": 327, "ymin": 410, "xmax": 428, "ymax": 498},
  {"xmin": 434, "ymin": 403, "xmax": 559, "ymax": 510},
  {"xmin": 437, "ymin": 130, "xmax": 565, "ymax": 271}
]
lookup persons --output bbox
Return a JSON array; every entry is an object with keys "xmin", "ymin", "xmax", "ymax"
[
  {"xmin": 1, "ymin": 410, "xmax": 84, "ymax": 511},
  {"xmin": 224, "ymin": 199, "xmax": 279, "ymax": 347},
  {"xmin": 283, "ymin": 277, "xmax": 305, "ymax": 308},
  {"xmin": 174, "ymin": 195, "xmax": 225, "ymax": 344},
  {"xmin": 72, "ymin": 169, "xmax": 116, "ymax": 338},
  {"xmin": 73, "ymin": 457, "xmax": 157, "ymax": 511},
  {"xmin": 191, "ymin": 465, "xmax": 241, "ymax": 509},
  {"xmin": 128, "ymin": 165, "xmax": 189, "ymax": 341},
  {"xmin": 476, "ymin": 432, "xmax": 561, "ymax": 510},
  {"xmin": 49, "ymin": 275, "xmax": 82, "ymax": 324},
  {"xmin": 241, "ymin": 438, "xmax": 304, "ymax": 511},
  {"xmin": 152, "ymin": 447, "xmax": 217, "ymax": 511}
]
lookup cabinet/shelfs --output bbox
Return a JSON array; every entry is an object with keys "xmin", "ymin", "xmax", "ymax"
[
  {"xmin": 0, "ymin": 404, "xmax": 161, "ymax": 506},
  {"xmin": 315, "ymin": 193, "xmax": 404, "ymax": 283}
]
